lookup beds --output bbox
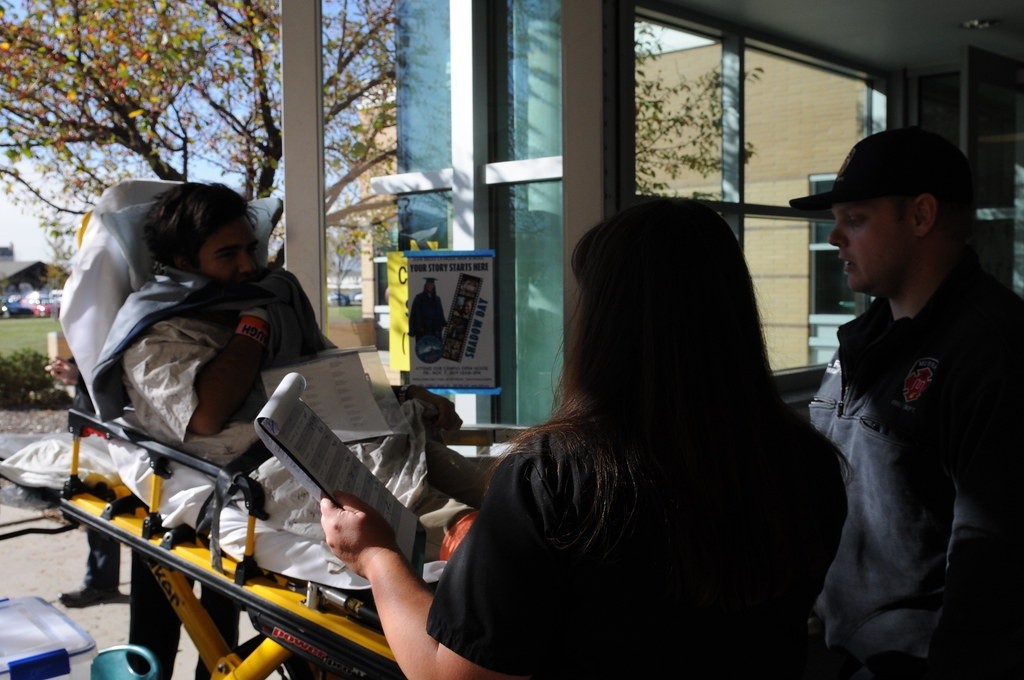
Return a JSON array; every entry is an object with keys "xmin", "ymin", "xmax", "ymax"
[{"xmin": 0, "ymin": 178, "xmax": 531, "ymax": 680}]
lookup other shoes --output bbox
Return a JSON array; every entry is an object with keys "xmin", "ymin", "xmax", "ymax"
[{"xmin": 60, "ymin": 586, "xmax": 120, "ymax": 608}]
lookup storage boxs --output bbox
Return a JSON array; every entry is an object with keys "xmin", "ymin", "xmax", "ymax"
[{"xmin": 0, "ymin": 596, "xmax": 97, "ymax": 680}]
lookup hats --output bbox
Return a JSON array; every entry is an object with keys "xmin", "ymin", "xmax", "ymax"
[{"xmin": 790, "ymin": 125, "xmax": 974, "ymax": 211}]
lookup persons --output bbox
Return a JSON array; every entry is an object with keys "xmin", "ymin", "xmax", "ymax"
[
  {"xmin": 444, "ymin": 280, "xmax": 478, "ymax": 355},
  {"xmin": 318, "ymin": 197, "xmax": 847, "ymax": 679},
  {"xmin": 791, "ymin": 126, "xmax": 1024, "ymax": 679},
  {"xmin": 409, "ymin": 277, "xmax": 448, "ymax": 343},
  {"xmin": 45, "ymin": 355, "xmax": 122, "ymax": 607},
  {"xmin": 121, "ymin": 181, "xmax": 420, "ymax": 443}
]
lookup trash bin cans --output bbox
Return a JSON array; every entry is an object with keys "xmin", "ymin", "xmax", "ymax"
[{"xmin": 47, "ymin": 330, "xmax": 73, "ymax": 363}]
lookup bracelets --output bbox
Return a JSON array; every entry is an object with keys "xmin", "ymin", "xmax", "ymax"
[
  {"xmin": 236, "ymin": 306, "xmax": 270, "ymax": 344},
  {"xmin": 399, "ymin": 382, "xmax": 414, "ymax": 398}
]
